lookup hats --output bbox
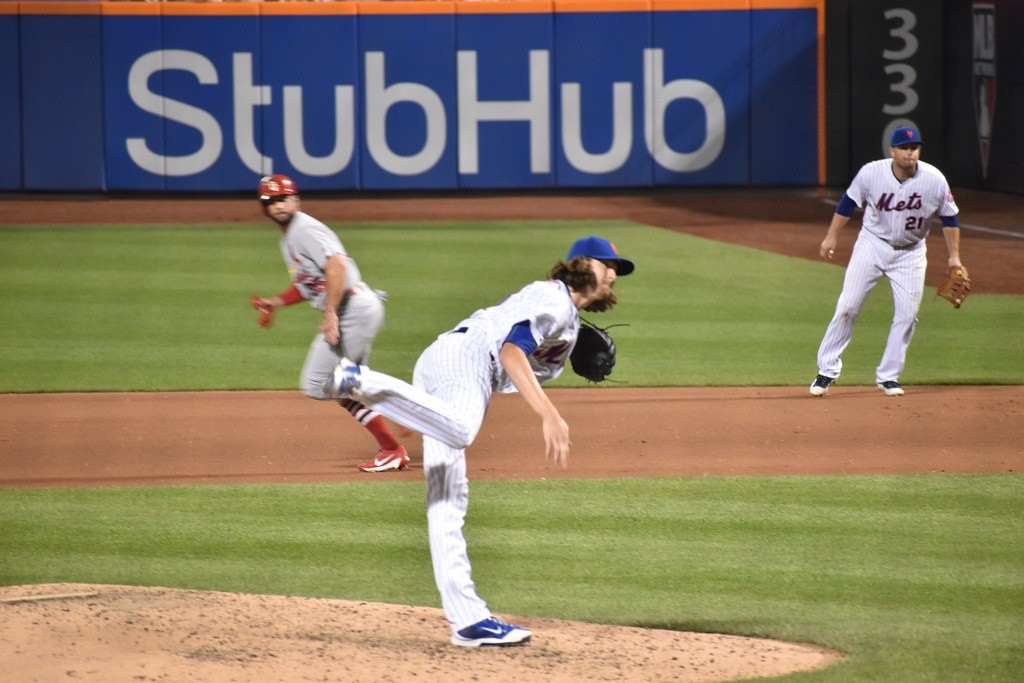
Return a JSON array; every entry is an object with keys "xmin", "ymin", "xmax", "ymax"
[
  {"xmin": 890, "ymin": 127, "xmax": 924, "ymax": 146},
  {"xmin": 567, "ymin": 236, "xmax": 635, "ymax": 275}
]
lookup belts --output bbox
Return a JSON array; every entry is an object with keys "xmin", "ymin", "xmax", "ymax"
[
  {"xmin": 345, "ymin": 285, "xmax": 364, "ymax": 297},
  {"xmin": 450, "ymin": 326, "xmax": 495, "ymax": 360}
]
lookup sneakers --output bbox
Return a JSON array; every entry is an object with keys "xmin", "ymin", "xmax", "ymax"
[
  {"xmin": 451, "ymin": 616, "xmax": 532, "ymax": 647},
  {"xmin": 358, "ymin": 444, "xmax": 410, "ymax": 472},
  {"xmin": 810, "ymin": 374, "xmax": 834, "ymax": 396},
  {"xmin": 877, "ymin": 381, "xmax": 904, "ymax": 396},
  {"xmin": 323, "ymin": 356, "xmax": 362, "ymax": 397}
]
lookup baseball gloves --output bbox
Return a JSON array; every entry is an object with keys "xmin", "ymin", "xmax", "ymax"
[
  {"xmin": 570, "ymin": 314, "xmax": 634, "ymax": 389},
  {"xmin": 936, "ymin": 265, "xmax": 972, "ymax": 310}
]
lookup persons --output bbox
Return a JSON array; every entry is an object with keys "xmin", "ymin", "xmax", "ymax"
[
  {"xmin": 808, "ymin": 121, "xmax": 968, "ymax": 399},
  {"xmin": 248, "ymin": 172, "xmax": 410, "ymax": 474},
  {"xmin": 324, "ymin": 235, "xmax": 636, "ymax": 648}
]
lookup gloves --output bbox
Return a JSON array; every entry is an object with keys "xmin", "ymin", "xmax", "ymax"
[{"xmin": 251, "ymin": 295, "xmax": 275, "ymax": 327}]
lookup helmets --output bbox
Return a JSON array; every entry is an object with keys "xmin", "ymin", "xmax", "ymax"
[{"xmin": 257, "ymin": 173, "xmax": 298, "ymax": 199}]
